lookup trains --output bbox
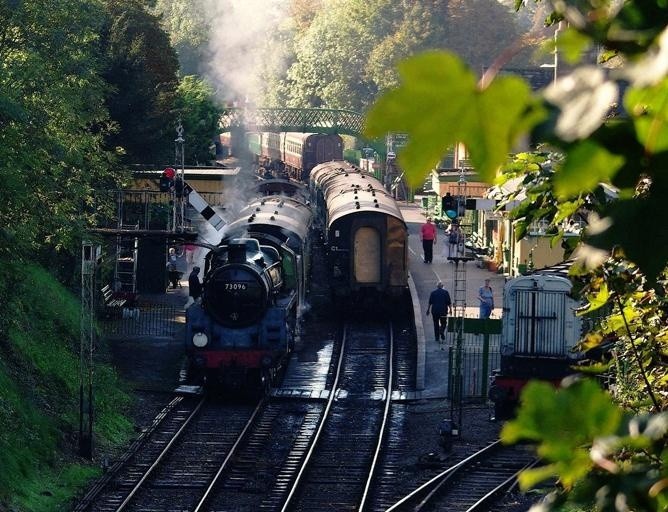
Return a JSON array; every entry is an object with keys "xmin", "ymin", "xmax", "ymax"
[
  {"xmin": 187, "ymin": 156, "xmax": 314, "ymax": 398},
  {"xmin": 488, "ymin": 256, "xmax": 635, "ymax": 419},
  {"xmin": 216, "ymin": 125, "xmax": 412, "ymax": 306}
]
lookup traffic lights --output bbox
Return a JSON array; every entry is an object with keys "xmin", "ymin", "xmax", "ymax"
[
  {"xmin": 440, "ymin": 191, "xmax": 467, "ymax": 220},
  {"xmin": 160, "ymin": 168, "xmax": 175, "ymax": 191}
]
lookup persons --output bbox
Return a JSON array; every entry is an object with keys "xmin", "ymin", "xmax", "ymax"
[
  {"xmin": 168, "ymin": 248, "xmax": 177, "ymax": 270},
  {"xmin": 425, "ymin": 279, "xmax": 453, "ymax": 343},
  {"xmin": 418, "ymin": 215, "xmax": 437, "ymax": 265},
  {"xmin": 186, "ymin": 265, "xmax": 205, "ymax": 303},
  {"xmin": 445, "ymin": 220, "xmax": 461, "ymax": 265},
  {"xmin": 476, "ymin": 277, "xmax": 495, "ymax": 319}
]
